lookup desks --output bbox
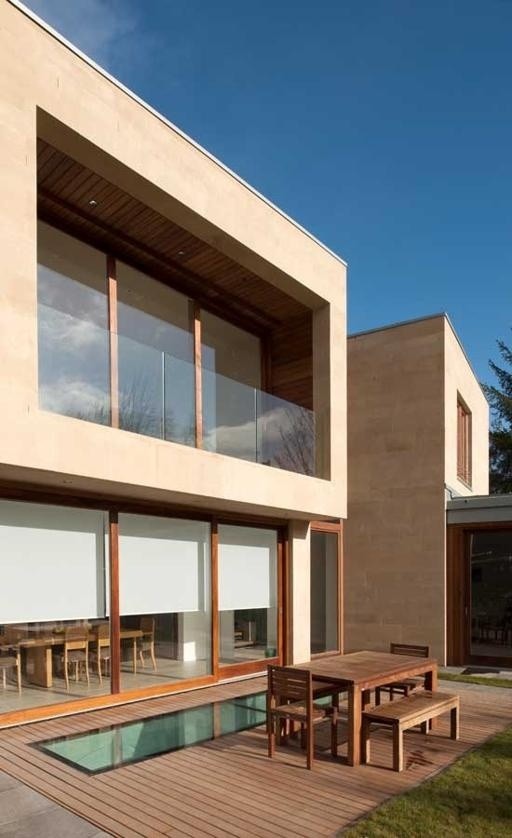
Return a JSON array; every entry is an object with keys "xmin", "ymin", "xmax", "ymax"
[{"xmin": 281, "ymin": 650, "xmax": 437, "ymax": 767}]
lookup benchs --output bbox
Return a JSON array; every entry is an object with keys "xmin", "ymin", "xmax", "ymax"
[
  {"xmin": 290, "ymin": 678, "xmax": 341, "ymax": 736},
  {"xmin": 361, "ymin": 690, "xmax": 459, "ymax": 771}
]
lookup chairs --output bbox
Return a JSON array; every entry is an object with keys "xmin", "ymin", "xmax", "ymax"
[
  {"xmin": 265, "ymin": 663, "xmax": 339, "ymax": 771},
  {"xmin": 375, "ymin": 643, "xmax": 428, "ymax": 705},
  {"xmin": 0, "ymin": 617, "xmax": 158, "ymax": 693}
]
